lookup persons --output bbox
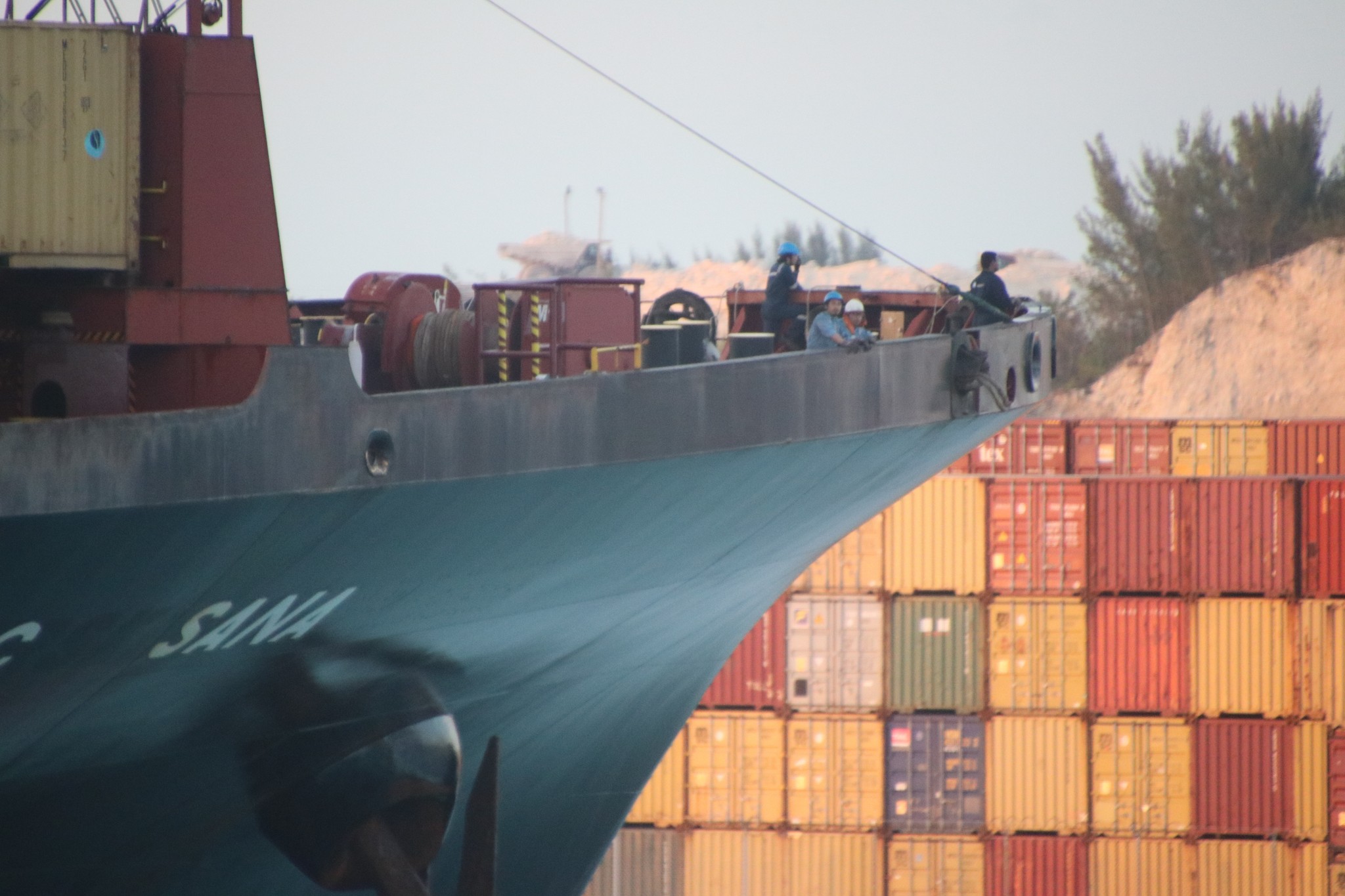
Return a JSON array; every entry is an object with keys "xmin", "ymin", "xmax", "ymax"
[
  {"xmin": 842, "ymin": 298, "xmax": 868, "ymax": 337},
  {"xmin": 760, "ymin": 241, "xmax": 807, "ymax": 350},
  {"xmin": 805, "ymin": 290, "xmax": 871, "ymax": 353},
  {"xmin": 969, "ymin": 252, "xmax": 1022, "ymax": 327}
]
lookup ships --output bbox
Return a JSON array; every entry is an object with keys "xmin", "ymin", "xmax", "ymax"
[{"xmin": 0, "ymin": 0, "xmax": 1070, "ymax": 895}]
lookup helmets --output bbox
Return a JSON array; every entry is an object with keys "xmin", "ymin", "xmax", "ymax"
[
  {"xmin": 845, "ymin": 299, "xmax": 865, "ymax": 313},
  {"xmin": 778, "ymin": 242, "xmax": 800, "ymax": 256},
  {"xmin": 824, "ymin": 292, "xmax": 843, "ymax": 303}
]
